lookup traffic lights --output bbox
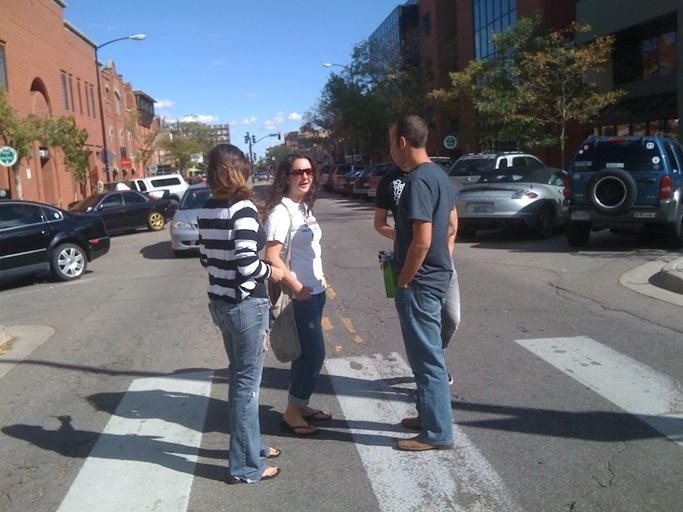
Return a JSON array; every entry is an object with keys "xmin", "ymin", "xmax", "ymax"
[
  {"xmin": 251, "ymin": 135, "xmax": 256, "ymax": 143},
  {"xmin": 244, "ymin": 136, "xmax": 247, "ymax": 144},
  {"xmin": 254, "ymin": 153, "xmax": 257, "ymax": 160},
  {"xmin": 278, "ymin": 133, "xmax": 281, "ymax": 140},
  {"xmin": 246, "ymin": 132, "xmax": 249, "ymax": 140}
]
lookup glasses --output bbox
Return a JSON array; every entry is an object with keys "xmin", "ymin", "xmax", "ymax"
[{"xmin": 287, "ymin": 168, "xmax": 316, "ymax": 177}]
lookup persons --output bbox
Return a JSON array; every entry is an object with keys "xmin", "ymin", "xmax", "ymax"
[
  {"xmin": 195, "ymin": 143, "xmax": 286, "ymax": 486},
  {"xmin": 372, "ymin": 123, "xmax": 462, "ymax": 386},
  {"xmin": 258, "ymin": 153, "xmax": 337, "ymax": 439},
  {"xmin": 391, "ymin": 115, "xmax": 455, "ymax": 452}
]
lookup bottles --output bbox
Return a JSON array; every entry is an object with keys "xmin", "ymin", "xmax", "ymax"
[{"xmin": 380, "ymin": 255, "xmax": 398, "ymax": 299}]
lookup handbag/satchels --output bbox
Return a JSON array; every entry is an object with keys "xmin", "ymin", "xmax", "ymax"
[{"xmin": 264, "ymin": 278, "xmax": 302, "ymax": 362}]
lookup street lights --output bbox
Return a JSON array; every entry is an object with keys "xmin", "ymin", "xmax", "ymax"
[
  {"xmin": 93, "ymin": 33, "xmax": 146, "ymax": 183},
  {"xmin": 322, "ymin": 63, "xmax": 358, "ymax": 153},
  {"xmin": 175, "ymin": 113, "xmax": 196, "ymax": 174}
]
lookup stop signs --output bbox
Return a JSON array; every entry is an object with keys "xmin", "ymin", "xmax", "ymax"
[{"xmin": 120, "ymin": 158, "xmax": 131, "ymax": 167}]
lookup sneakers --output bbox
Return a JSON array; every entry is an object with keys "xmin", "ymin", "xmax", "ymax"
[
  {"xmin": 402, "ymin": 418, "xmax": 423, "ymax": 430},
  {"xmin": 397, "ymin": 434, "xmax": 451, "ymax": 450}
]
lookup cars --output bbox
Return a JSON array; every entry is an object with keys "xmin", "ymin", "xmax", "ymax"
[
  {"xmin": 0, "ymin": 173, "xmax": 211, "ymax": 283},
  {"xmin": 255, "ymin": 173, "xmax": 273, "ymax": 181}
]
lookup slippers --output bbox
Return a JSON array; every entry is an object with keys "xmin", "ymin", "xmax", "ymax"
[
  {"xmin": 227, "ymin": 467, "xmax": 280, "ymax": 485},
  {"xmin": 280, "ymin": 418, "xmax": 318, "ymax": 437},
  {"xmin": 306, "ymin": 408, "xmax": 332, "ymax": 421},
  {"xmin": 269, "ymin": 447, "xmax": 281, "ymax": 458}
]
